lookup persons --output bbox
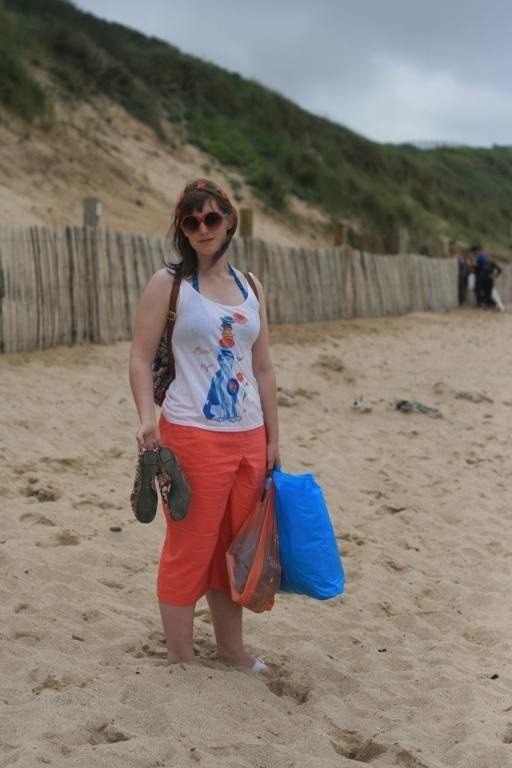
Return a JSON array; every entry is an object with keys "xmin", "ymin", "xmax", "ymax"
[
  {"xmin": 129, "ymin": 179, "xmax": 281, "ymax": 680},
  {"xmin": 457, "ymin": 241, "xmax": 500, "ymax": 307}
]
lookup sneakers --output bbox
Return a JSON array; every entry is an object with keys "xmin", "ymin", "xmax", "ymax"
[
  {"xmin": 151, "ymin": 444, "xmax": 194, "ymax": 522},
  {"xmin": 127, "ymin": 448, "xmax": 160, "ymax": 525}
]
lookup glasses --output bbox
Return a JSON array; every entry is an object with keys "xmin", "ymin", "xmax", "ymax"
[{"xmin": 179, "ymin": 211, "xmax": 230, "ymax": 234}]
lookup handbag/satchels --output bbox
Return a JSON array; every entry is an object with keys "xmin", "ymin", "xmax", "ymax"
[
  {"xmin": 263, "ymin": 469, "xmax": 349, "ymax": 598},
  {"xmin": 150, "ymin": 336, "xmax": 175, "ymax": 406}
]
